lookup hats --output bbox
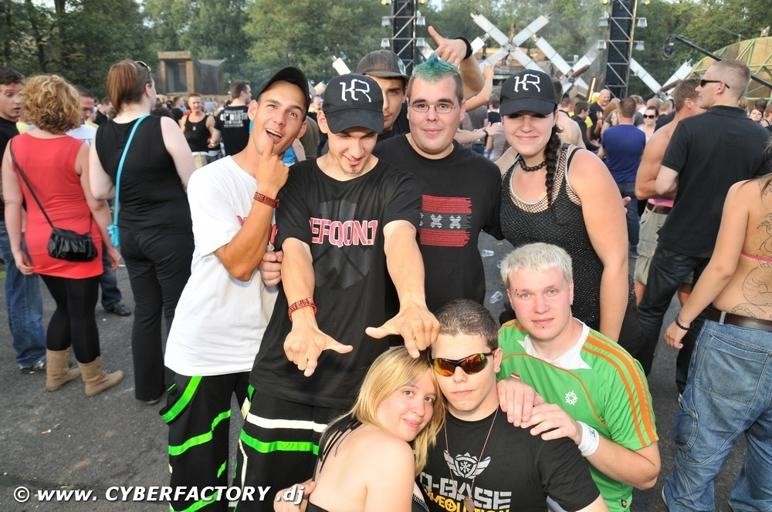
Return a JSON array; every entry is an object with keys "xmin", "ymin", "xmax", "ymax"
[
  {"xmin": 355, "ymin": 47, "xmax": 410, "ymax": 81},
  {"xmin": 322, "ymin": 72, "xmax": 386, "ymax": 135},
  {"xmin": 252, "ymin": 66, "xmax": 311, "ymax": 111},
  {"xmin": 498, "ymin": 69, "xmax": 558, "ymax": 117}
]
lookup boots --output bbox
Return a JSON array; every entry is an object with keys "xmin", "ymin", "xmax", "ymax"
[
  {"xmin": 44, "ymin": 344, "xmax": 82, "ymax": 392},
  {"xmin": 76, "ymin": 355, "xmax": 124, "ymax": 397}
]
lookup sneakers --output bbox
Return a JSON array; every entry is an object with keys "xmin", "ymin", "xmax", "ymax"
[
  {"xmin": 103, "ymin": 301, "xmax": 132, "ymax": 316},
  {"xmin": 17, "ymin": 357, "xmax": 47, "ymax": 376}
]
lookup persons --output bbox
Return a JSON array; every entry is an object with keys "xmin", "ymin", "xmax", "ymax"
[{"xmin": 0, "ymin": 25, "xmax": 772, "ymax": 512}]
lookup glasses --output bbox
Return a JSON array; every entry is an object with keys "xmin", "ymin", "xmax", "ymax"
[
  {"xmin": 700, "ymin": 77, "xmax": 731, "ymax": 89},
  {"xmin": 430, "ymin": 352, "xmax": 495, "ymax": 378},
  {"xmin": 408, "ymin": 100, "xmax": 460, "ymax": 114},
  {"xmin": 642, "ymin": 113, "xmax": 656, "ymax": 119}
]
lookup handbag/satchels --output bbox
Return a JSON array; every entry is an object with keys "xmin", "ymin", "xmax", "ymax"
[
  {"xmin": 106, "ymin": 224, "xmax": 120, "ymax": 252},
  {"xmin": 47, "ymin": 227, "xmax": 99, "ymax": 262}
]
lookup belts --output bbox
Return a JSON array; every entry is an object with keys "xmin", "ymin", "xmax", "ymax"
[{"xmin": 701, "ymin": 307, "xmax": 772, "ymax": 333}]
